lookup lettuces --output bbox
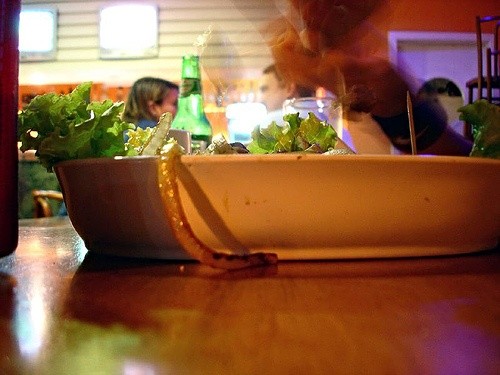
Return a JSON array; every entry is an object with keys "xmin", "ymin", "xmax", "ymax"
[{"xmin": 17, "ymin": 80, "xmax": 500, "ymax": 175}]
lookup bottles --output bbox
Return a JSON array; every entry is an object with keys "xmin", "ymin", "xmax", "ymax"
[{"xmin": 172, "ymin": 55, "xmax": 213, "ymax": 149}]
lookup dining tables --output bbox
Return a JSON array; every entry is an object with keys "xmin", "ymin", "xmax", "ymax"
[{"xmin": 0, "ymin": 215, "xmax": 500, "ymax": 375}]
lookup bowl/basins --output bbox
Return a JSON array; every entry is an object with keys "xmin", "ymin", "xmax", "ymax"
[{"xmin": 58, "ymin": 153, "xmax": 500, "ymax": 260}]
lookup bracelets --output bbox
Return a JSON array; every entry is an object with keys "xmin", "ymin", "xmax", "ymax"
[{"xmin": 368, "ymin": 94, "xmax": 452, "ymax": 154}]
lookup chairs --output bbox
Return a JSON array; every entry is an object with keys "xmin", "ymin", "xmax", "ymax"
[
  {"xmin": 466, "ymin": 14, "xmax": 500, "ymax": 107},
  {"xmin": 31, "ymin": 191, "xmax": 64, "ymax": 217}
]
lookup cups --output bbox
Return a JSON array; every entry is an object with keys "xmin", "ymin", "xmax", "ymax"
[
  {"xmin": 225, "ymin": 103, "xmax": 268, "ymax": 144},
  {"xmin": 282, "ymin": 97, "xmax": 342, "ymax": 141}
]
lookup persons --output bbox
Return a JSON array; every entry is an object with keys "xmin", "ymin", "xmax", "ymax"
[
  {"xmin": 56, "ymin": 77, "xmax": 181, "ymax": 217},
  {"xmin": 316, "ymin": 48, "xmax": 478, "ymax": 158},
  {"xmin": 255, "ymin": 64, "xmax": 330, "ymax": 132}
]
